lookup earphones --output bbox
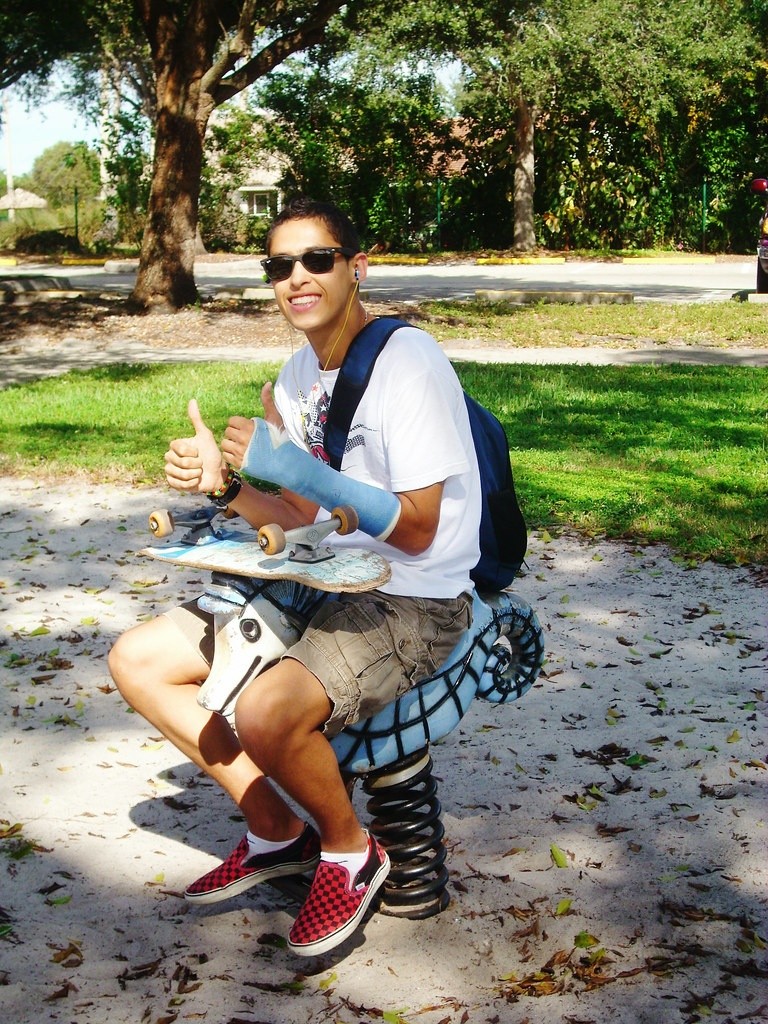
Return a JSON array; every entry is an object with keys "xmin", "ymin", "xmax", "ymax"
[{"xmin": 355, "ymin": 269, "xmax": 359, "ymax": 281}]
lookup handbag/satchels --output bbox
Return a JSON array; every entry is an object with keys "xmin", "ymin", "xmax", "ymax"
[{"xmin": 463, "ymin": 387, "xmax": 529, "ymax": 593}]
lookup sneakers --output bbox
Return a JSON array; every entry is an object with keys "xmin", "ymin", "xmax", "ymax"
[
  {"xmin": 184, "ymin": 820, "xmax": 322, "ymax": 907},
  {"xmin": 287, "ymin": 829, "xmax": 391, "ymax": 958}
]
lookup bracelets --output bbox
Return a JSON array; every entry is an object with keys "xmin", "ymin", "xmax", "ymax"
[
  {"xmin": 203, "ymin": 467, "xmax": 235, "ymax": 498},
  {"xmin": 207, "ymin": 471, "xmax": 244, "ymax": 511}
]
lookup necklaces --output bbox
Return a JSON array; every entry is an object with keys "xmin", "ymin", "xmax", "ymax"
[{"xmin": 364, "ymin": 310, "xmax": 369, "ymax": 327}]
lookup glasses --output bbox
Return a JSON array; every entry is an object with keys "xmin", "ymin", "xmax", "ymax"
[{"xmin": 259, "ymin": 246, "xmax": 355, "ymax": 283}]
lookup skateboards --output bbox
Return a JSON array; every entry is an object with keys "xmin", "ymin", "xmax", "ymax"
[{"xmin": 144, "ymin": 502, "xmax": 392, "ymax": 595}]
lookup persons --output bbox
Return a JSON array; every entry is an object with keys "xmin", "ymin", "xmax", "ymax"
[{"xmin": 106, "ymin": 197, "xmax": 484, "ymax": 958}]
